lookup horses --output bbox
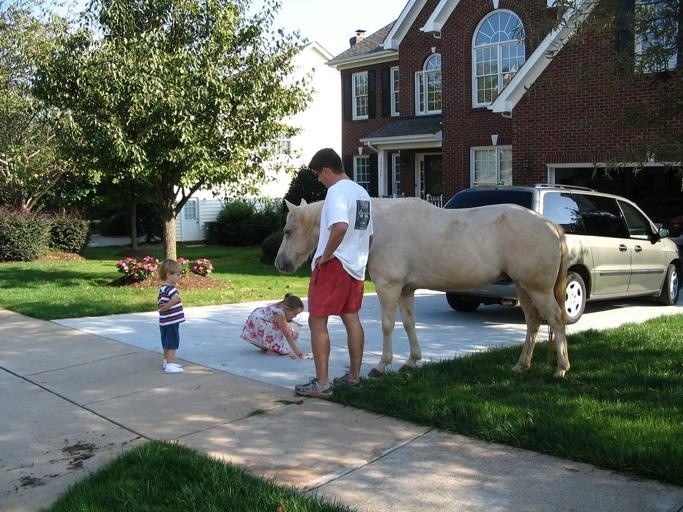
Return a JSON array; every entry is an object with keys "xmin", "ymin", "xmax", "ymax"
[{"xmin": 274, "ymin": 198, "xmax": 572, "ymax": 382}]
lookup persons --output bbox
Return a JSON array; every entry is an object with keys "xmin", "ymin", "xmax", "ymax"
[
  {"xmin": 158, "ymin": 259, "xmax": 186, "ymax": 372},
  {"xmin": 294, "ymin": 149, "xmax": 374, "ymax": 395},
  {"xmin": 240, "ymin": 293, "xmax": 309, "ymax": 360}
]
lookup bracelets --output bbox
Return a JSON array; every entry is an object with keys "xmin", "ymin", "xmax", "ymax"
[{"xmin": 299, "ymin": 352, "xmax": 305, "ymax": 357}]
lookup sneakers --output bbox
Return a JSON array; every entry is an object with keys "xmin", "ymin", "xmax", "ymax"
[
  {"xmin": 294, "ymin": 379, "xmax": 332, "ymax": 397},
  {"xmin": 333, "ymin": 374, "xmax": 361, "ymax": 385},
  {"xmin": 162, "ymin": 360, "xmax": 183, "ymax": 373}
]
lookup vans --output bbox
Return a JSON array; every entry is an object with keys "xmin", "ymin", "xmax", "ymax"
[{"xmin": 434, "ymin": 178, "xmax": 679, "ymax": 325}]
